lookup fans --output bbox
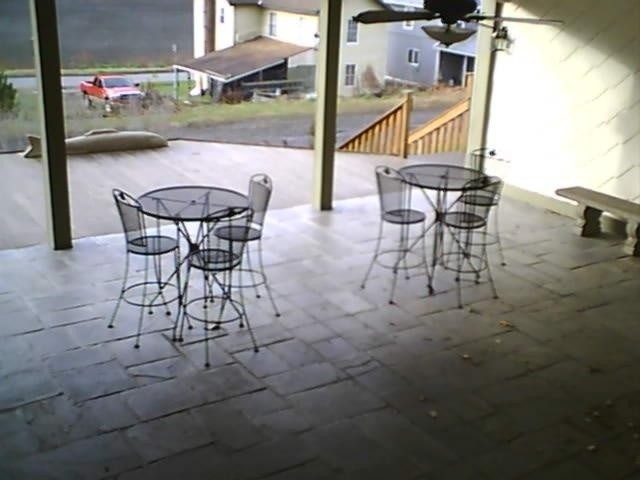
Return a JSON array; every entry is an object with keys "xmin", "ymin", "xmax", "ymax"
[{"xmin": 350, "ymin": 0, "xmax": 565, "ymax": 28}]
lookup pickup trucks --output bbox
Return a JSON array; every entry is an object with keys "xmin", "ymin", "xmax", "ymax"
[{"xmin": 79, "ymin": 73, "xmax": 145, "ymax": 112}]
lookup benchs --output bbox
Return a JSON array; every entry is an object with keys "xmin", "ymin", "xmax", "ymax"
[{"xmin": 555, "ymin": 184, "xmax": 639, "ymax": 256}]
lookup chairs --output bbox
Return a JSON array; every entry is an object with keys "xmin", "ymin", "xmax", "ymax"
[
  {"xmin": 107, "ymin": 174, "xmax": 281, "ymax": 369},
  {"xmin": 359, "ymin": 146, "xmax": 514, "ymax": 313}
]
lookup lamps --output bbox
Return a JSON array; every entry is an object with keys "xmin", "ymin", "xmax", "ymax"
[{"xmin": 420, "ymin": 15, "xmax": 477, "ymax": 47}]
